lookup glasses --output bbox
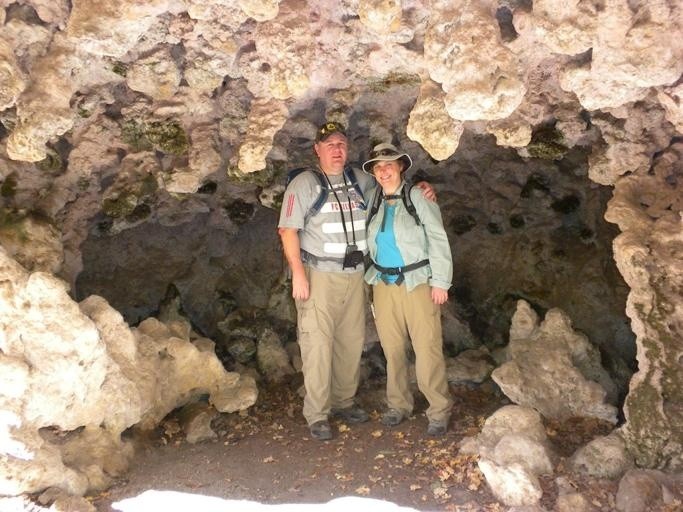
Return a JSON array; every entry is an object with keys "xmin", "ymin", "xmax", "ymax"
[{"xmin": 369, "ymin": 149, "xmax": 399, "ymax": 158}]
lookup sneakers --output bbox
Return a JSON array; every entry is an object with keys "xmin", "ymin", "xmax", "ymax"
[
  {"xmin": 427, "ymin": 419, "xmax": 449, "ymax": 435},
  {"xmin": 309, "ymin": 421, "xmax": 332, "ymax": 440},
  {"xmin": 330, "ymin": 404, "xmax": 368, "ymax": 422},
  {"xmin": 382, "ymin": 409, "xmax": 412, "ymax": 425}
]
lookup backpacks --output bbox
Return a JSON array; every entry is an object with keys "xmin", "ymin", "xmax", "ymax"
[{"xmin": 286, "ymin": 164, "xmax": 366, "ymax": 225}]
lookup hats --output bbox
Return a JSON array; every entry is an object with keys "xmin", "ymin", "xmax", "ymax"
[
  {"xmin": 315, "ymin": 122, "xmax": 345, "ymax": 144},
  {"xmin": 362, "ymin": 143, "xmax": 412, "ymax": 177}
]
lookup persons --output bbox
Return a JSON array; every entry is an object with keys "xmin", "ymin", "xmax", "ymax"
[
  {"xmin": 363, "ymin": 143, "xmax": 454, "ymax": 436},
  {"xmin": 278, "ymin": 122, "xmax": 437, "ymax": 440}
]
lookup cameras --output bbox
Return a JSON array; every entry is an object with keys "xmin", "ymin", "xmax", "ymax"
[{"xmin": 343, "ymin": 245, "xmax": 364, "ymax": 267}]
